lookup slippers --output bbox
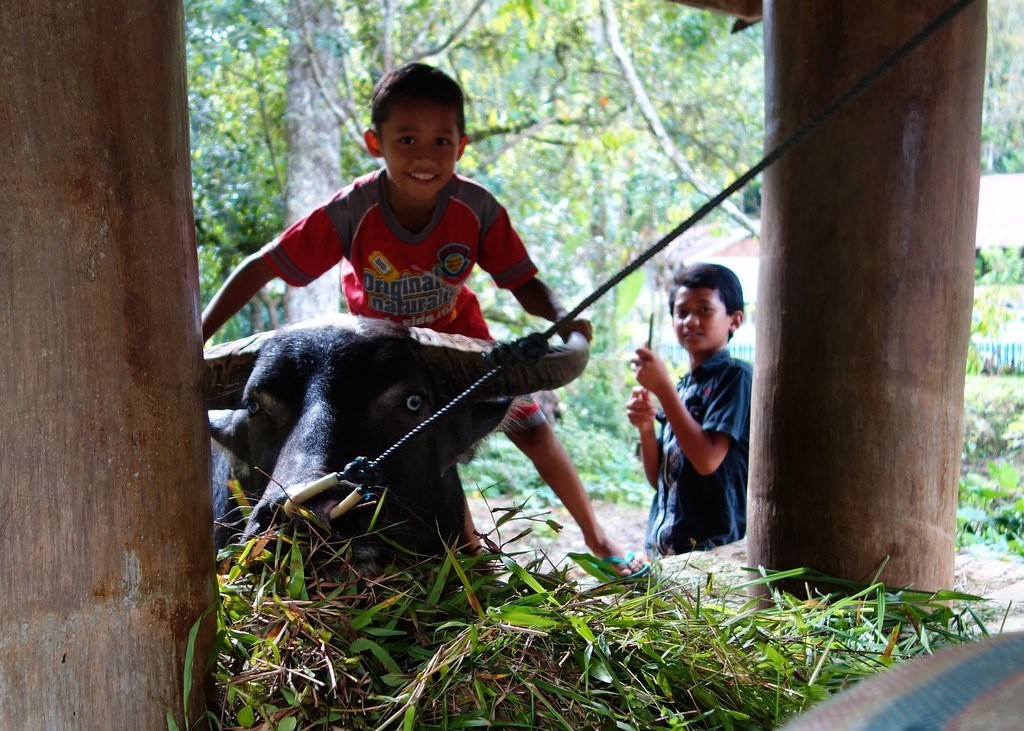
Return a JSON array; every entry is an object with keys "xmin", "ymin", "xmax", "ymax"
[{"xmin": 590, "ymin": 553, "xmax": 650, "ymax": 584}]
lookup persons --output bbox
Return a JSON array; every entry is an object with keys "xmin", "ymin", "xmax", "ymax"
[
  {"xmin": 199, "ymin": 62, "xmax": 649, "ymax": 581},
  {"xmin": 627, "ymin": 262, "xmax": 754, "ymax": 559}
]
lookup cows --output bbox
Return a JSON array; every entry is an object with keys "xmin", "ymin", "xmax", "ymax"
[{"xmin": 205, "ymin": 312, "xmax": 589, "ymax": 572}]
